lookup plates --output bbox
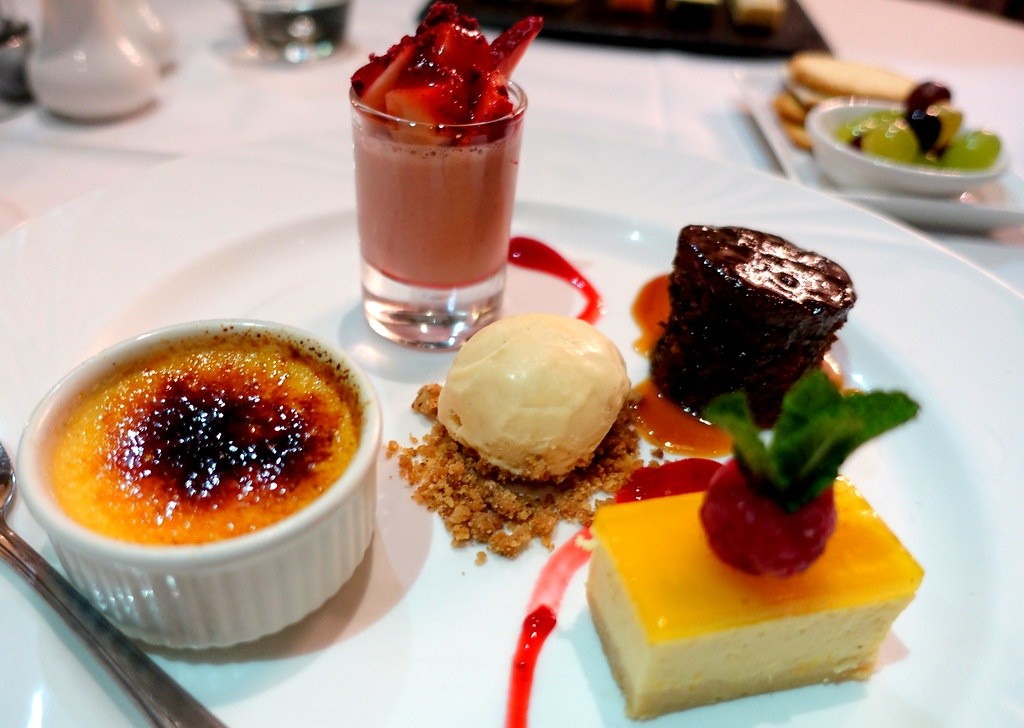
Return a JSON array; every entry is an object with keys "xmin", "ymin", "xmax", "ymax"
[
  {"xmin": 737, "ymin": 76, "xmax": 1024, "ymax": 230},
  {"xmin": 418, "ymin": 0, "xmax": 833, "ymax": 55},
  {"xmin": 1, "ymin": 116, "xmax": 1023, "ymax": 727}
]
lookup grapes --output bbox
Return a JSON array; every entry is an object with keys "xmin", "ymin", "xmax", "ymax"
[{"xmin": 836, "ymin": 80, "xmax": 1001, "ymax": 171}]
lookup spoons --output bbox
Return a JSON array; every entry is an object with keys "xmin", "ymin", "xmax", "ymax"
[{"xmin": 0, "ymin": 440, "xmax": 231, "ymax": 727}]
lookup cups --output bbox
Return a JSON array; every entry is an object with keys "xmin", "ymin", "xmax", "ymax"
[
  {"xmin": 17, "ymin": 320, "xmax": 382, "ymax": 647},
  {"xmin": 236, "ymin": 1, "xmax": 352, "ymax": 61},
  {"xmin": 348, "ymin": 77, "xmax": 529, "ymax": 347}
]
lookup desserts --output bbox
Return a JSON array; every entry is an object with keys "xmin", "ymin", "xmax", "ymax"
[{"xmin": 14, "ymin": 219, "xmax": 928, "ymax": 724}]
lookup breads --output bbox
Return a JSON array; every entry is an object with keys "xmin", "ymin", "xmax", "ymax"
[{"xmin": 773, "ymin": 50, "xmax": 917, "ymax": 149}]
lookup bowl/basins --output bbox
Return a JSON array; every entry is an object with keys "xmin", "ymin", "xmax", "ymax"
[{"xmin": 806, "ymin": 97, "xmax": 1010, "ymax": 196}]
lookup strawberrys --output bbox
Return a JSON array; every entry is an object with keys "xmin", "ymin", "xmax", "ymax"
[{"xmin": 348, "ymin": 0, "xmax": 544, "ymax": 149}]
